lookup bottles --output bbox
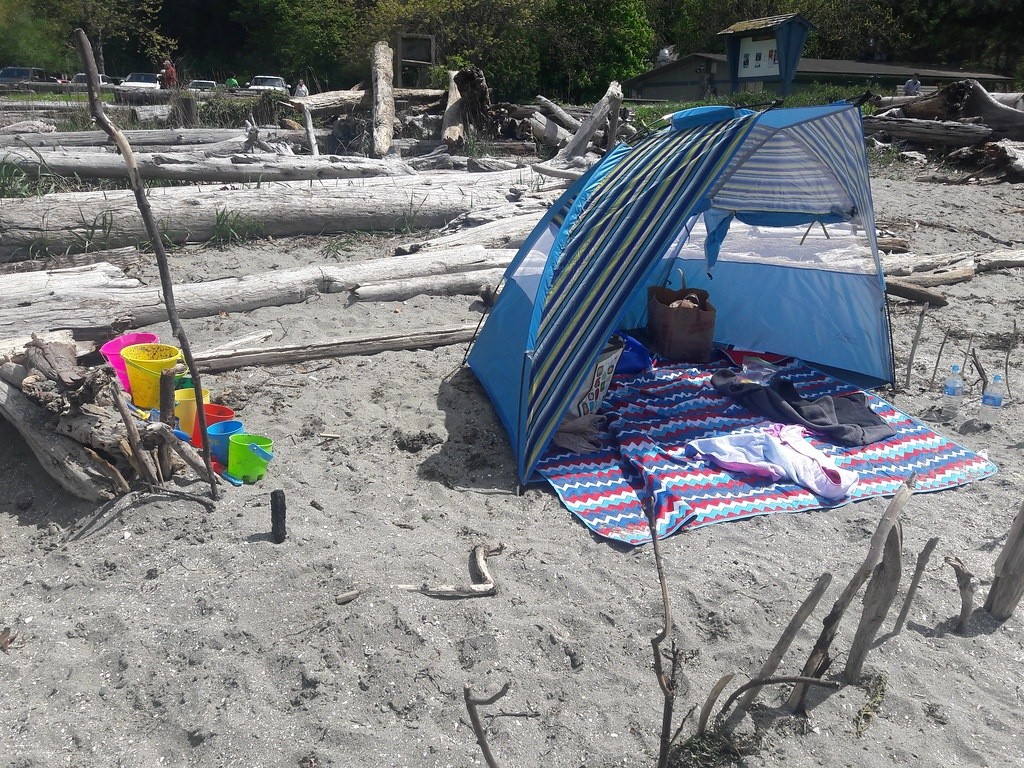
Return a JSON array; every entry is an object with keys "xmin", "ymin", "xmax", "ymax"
[
  {"xmin": 979, "ymin": 375, "xmax": 1005, "ymax": 426},
  {"xmin": 941, "ymin": 365, "xmax": 964, "ymax": 418}
]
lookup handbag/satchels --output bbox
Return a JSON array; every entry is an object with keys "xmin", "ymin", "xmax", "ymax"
[
  {"xmin": 613, "ymin": 331, "xmax": 653, "ymax": 375},
  {"xmin": 569, "ymin": 336, "xmax": 627, "ymax": 419},
  {"xmin": 646, "ymin": 268, "xmax": 716, "ymax": 364}
]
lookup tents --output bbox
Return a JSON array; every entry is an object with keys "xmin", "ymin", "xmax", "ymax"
[{"xmin": 462, "ymin": 91, "xmax": 897, "ymax": 495}]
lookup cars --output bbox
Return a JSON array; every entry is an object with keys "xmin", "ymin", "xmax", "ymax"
[
  {"xmin": 49, "ymin": 76, "xmax": 71, "ymax": 83},
  {"xmin": 67, "ymin": 73, "xmax": 115, "ymax": 86},
  {"xmin": 117, "ymin": 73, "xmax": 162, "ymax": 90},
  {"xmin": 186, "ymin": 80, "xmax": 218, "ymax": 94},
  {"xmin": 245, "ymin": 76, "xmax": 291, "ymax": 96}
]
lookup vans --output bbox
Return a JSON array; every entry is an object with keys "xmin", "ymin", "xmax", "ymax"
[{"xmin": 0, "ymin": 67, "xmax": 48, "ymax": 83}]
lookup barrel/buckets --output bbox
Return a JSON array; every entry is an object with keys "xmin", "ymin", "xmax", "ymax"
[
  {"xmin": 99, "ymin": 333, "xmax": 160, "ymax": 394},
  {"xmin": 174, "ymin": 377, "xmax": 196, "ymax": 390},
  {"xmin": 206, "ymin": 420, "xmax": 243, "ymax": 466},
  {"xmin": 228, "ymin": 433, "xmax": 273, "ymax": 484},
  {"xmin": 121, "ymin": 342, "xmax": 189, "ymax": 409},
  {"xmin": 172, "ymin": 388, "xmax": 211, "ymax": 438},
  {"xmin": 191, "ymin": 404, "xmax": 235, "ymax": 448}
]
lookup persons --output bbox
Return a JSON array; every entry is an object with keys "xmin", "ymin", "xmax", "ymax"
[
  {"xmin": 226, "ymin": 74, "xmax": 239, "ymax": 93},
  {"xmin": 904, "ymin": 73, "xmax": 920, "ymax": 96},
  {"xmin": 158, "ymin": 60, "xmax": 176, "ymax": 90},
  {"xmin": 295, "ymin": 79, "xmax": 308, "ymax": 97}
]
